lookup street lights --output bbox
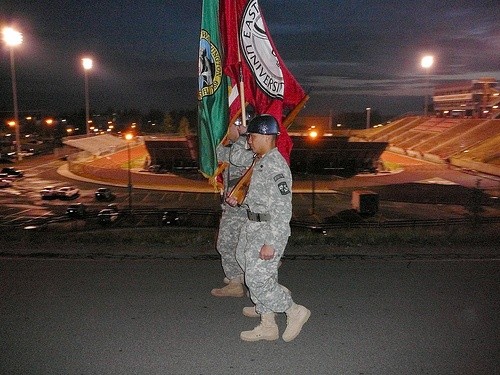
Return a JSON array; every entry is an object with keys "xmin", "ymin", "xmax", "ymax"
[
  {"xmin": 123, "ymin": 131, "xmax": 136, "ymax": 217},
  {"xmin": 309, "ymin": 129, "xmax": 319, "ymax": 214}
]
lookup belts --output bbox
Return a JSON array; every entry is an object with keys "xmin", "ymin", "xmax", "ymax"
[
  {"xmin": 246, "ymin": 209, "xmax": 267, "ymax": 222},
  {"xmin": 228, "ymin": 178, "xmax": 239, "ymax": 187}
]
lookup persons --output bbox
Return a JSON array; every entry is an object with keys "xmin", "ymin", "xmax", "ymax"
[
  {"xmin": 225, "ymin": 113, "xmax": 312, "ymax": 344},
  {"xmin": 211, "ymin": 105, "xmax": 257, "ymax": 298}
]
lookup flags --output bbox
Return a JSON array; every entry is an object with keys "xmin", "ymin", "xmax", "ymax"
[
  {"xmin": 196, "ymin": 1, "xmax": 225, "ymax": 176},
  {"xmin": 219, "ymin": 1, "xmax": 308, "ymax": 193}
]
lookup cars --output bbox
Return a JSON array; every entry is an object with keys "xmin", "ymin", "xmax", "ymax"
[
  {"xmin": 66, "ymin": 202, "xmax": 87, "ymax": 215},
  {"xmin": 2, "ymin": 166, "xmax": 24, "ymax": 178},
  {"xmin": 97, "ymin": 207, "xmax": 117, "ymax": 222},
  {"xmin": 57, "ymin": 184, "xmax": 80, "ymax": 199},
  {"xmin": 162, "ymin": 212, "xmax": 180, "ymax": 225},
  {"xmin": 41, "ymin": 185, "xmax": 57, "ymax": 198},
  {"xmin": 94, "ymin": 187, "xmax": 111, "ymax": 201}
]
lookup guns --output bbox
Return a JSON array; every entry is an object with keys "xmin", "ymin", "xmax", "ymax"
[{"xmin": 228, "ymin": 86, "xmax": 313, "ymax": 208}]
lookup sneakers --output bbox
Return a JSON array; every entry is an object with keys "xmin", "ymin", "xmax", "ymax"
[
  {"xmin": 224, "ymin": 274, "xmax": 244, "ymax": 286},
  {"xmin": 282, "ymin": 302, "xmax": 311, "ymax": 342},
  {"xmin": 240, "ymin": 311, "xmax": 280, "ymax": 342},
  {"xmin": 210, "ymin": 278, "xmax": 244, "ymax": 297},
  {"xmin": 243, "ymin": 306, "xmax": 260, "ymax": 318}
]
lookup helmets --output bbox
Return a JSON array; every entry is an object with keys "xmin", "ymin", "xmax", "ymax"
[
  {"xmin": 233, "ymin": 104, "xmax": 255, "ymax": 126},
  {"xmin": 244, "ymin": 114, "xmax": 281, "ymax": 135}
]
